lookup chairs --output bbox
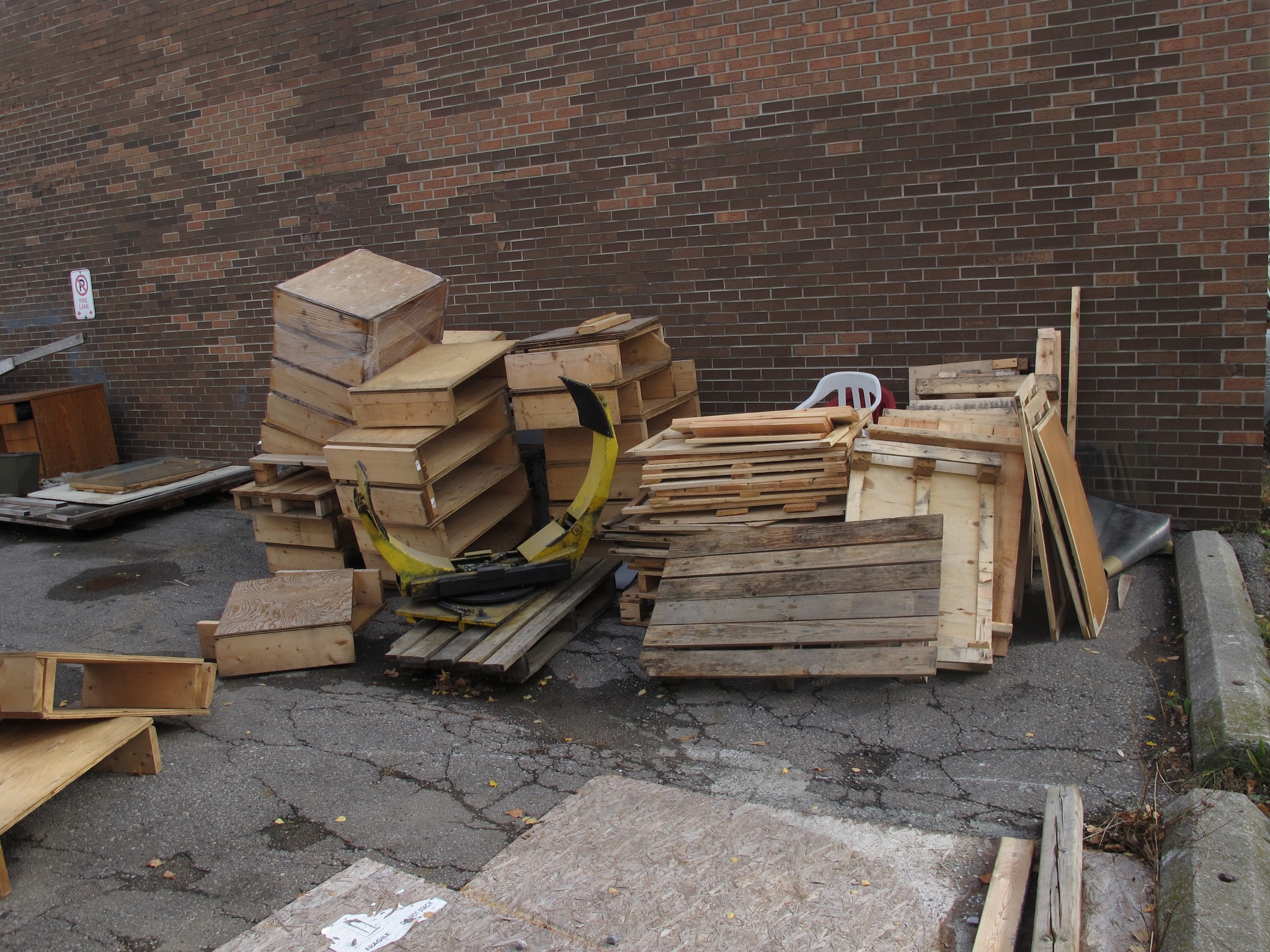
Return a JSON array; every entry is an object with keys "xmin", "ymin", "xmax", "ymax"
[
  {"xmin": 793, "ymin": 371, "xmax": 882, "ymax": 426},
  {"xmin": 825, "ymin": 386, "xmax": 895, "ymax": 425}
]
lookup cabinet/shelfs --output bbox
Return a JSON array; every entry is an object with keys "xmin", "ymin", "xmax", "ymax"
[{"xmin": 0, "ymin": 383, "xmax": 120, "ymax": 480}]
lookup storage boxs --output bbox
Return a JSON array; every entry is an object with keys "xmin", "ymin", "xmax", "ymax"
[{"xmin": 0, "ymin": 452, "xmax": 42, "ymax": 497}]
